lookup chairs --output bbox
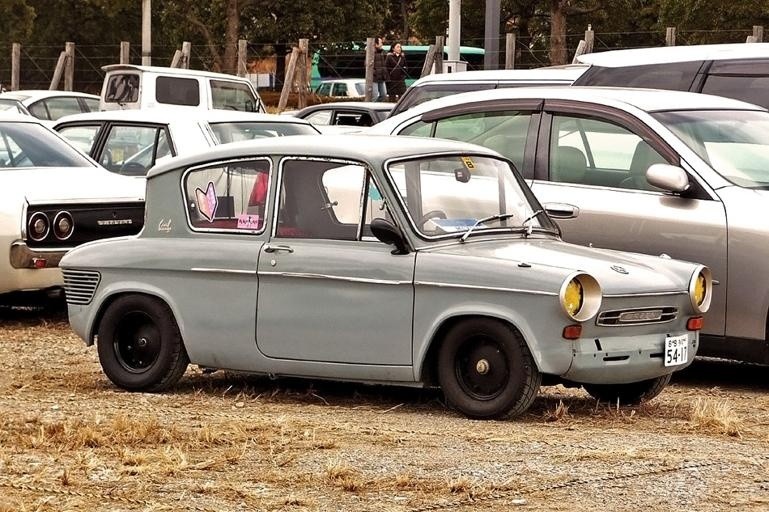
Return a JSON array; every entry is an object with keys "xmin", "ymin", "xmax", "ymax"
[
  {"xmin": 482, "ymin": 133, "xmax": 526, "ymax": 171},
  {"xmin": 551, "ymin": 138, "xmax": 674, "ymax": 192}
]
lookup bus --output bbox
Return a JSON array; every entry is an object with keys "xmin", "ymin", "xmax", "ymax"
[{"xmin": 309, "ymin": 42, "xmax": 487, "ymax": 95}]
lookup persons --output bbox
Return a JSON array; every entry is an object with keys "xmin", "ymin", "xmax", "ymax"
[
  {"xmin": 371, "ymin": 37, "xmax": 390, "ymax": 101},
  {"xmin": 386, "ymin": 41, "xmax": 408, "ymax": 101}
]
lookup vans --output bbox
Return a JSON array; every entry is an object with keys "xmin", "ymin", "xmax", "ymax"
[{"xmin": 97, "ymin": 61, "xmax": 270, "ymax": 119}]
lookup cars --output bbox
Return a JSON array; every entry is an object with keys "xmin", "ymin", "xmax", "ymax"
[
  {"xmin": 0, "ymin": 112, "xmax": 147, "ymax": 315},
  {"xmin": 296, "ymin": 101, "xmax": 397, "ymax": 126},
  {"xmin": 57, "ymin": 133, "xmax": 713, "ymax": 422},
  {"xmin": 313, "ymin": 77, "xmax": 385, "ymax": 101},
  {"xmin": 2, "ymin": 89, "xmax": 102, "ymax": 127},
  {"xmin": 52, "ymin": 108, "xmax": 322, "ymax": 178}
]
